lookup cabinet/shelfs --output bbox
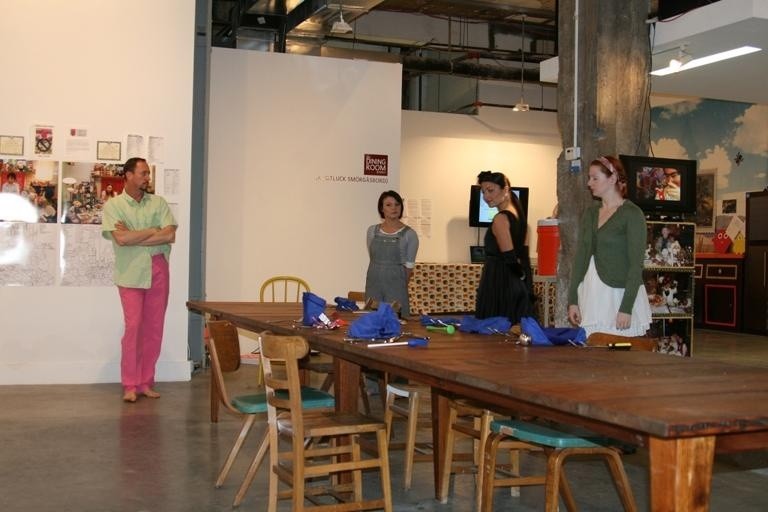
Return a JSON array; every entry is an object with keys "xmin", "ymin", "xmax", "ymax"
[{"xmin": 699, "ymin": 257, "xmax": 739, "ymax": 328}]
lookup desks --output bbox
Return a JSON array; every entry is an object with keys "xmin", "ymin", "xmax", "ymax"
[
  {"xmin": 186, "ymin": 299, "xmax": 521, "ymax": 510},
  {"xmin": 336, "ymin": 334, "xmax": 768, "ymax": 512}
]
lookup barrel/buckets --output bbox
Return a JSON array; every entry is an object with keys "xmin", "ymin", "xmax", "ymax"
[{"xmin": 537, "ymin": 219, "xmax": 560, "ymax": 275}]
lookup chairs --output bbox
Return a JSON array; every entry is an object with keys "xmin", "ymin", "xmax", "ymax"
[
  {"xmin": 383, "ymin": 316, "xmax": 509, "ymax": 492},
  {"xmin": 253, "ymin": 334, "xmax": 394, "ymax": 510},
  {"xmin": 483, "ymin": 333, "xmax": 656, "ymax": 511},
  {"xmin": 260, "ymin": 276, "xmax": 309, "ymax": 302},
  {"xmin": 435, "ymin": 314, "xmax": 584, "ymax": 503},
  {"xmin": 199, "ymin": 320, "xmax": 333, "ymax": 505}
]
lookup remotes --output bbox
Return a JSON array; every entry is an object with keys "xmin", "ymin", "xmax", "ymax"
[{"xmin": 663, "ymin": 217, "xmax": 684, "ymax": 222}]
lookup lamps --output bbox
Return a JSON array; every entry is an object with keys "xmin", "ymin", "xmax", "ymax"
[
  {"xmin": 327, "ymin": 8, "xmax": 352, "ymax": 35},
  {"xmin": 513, "ymin": 8, "xmax": 529, "ymax": 116},
  {"xmin": 666, "ymin": 48, "xmax": 692, "ymax": 73}
]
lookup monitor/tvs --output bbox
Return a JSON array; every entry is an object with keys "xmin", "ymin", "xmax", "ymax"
[
  {"xmin": 619, "ymin": 154, "xmax": 697, "ymax": 221},
  {"xmin": 469, "ymin": 185, "xmax": 528, "ymax": 228}
]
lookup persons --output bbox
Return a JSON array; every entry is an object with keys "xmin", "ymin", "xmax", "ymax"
[
  {"xmin": 567, "ymin": 153, "xmax": 653, "ymax": 339},
  {"xmin": 477, "ymin": 171, "xmax": 540, "ymax": 326},
  {"xmin": 3, "ymin": 171, "xmax": 20, "ymax": 193},
  {"xmin": 100, "ymin": 182, "xmax": 116, "ymax": 204},
  {"xmin": 100, "ymin": 158, "xmax": 177, "ymax": 402},
  {"xmin": 642, "ymin": 224, "xmax": 688, "ymax": 357},
  {"xmin": 364, "ymin": 191, "xmax": 419, "ymax": 400},
  {"xmin": 654, "ymin": 165, "xmax": 680, "ymax": 201}
]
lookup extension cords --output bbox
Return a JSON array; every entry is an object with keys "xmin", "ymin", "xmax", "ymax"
[{"xmin": 645, "ymin": 16, "xmax": 658, "ymax": 23}]
